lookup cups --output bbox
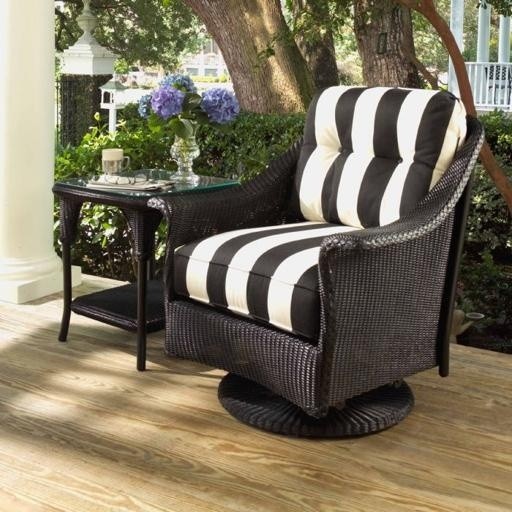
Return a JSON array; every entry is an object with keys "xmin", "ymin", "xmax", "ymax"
[{"xmin": 101, "ymin": 148, "xmax": 130, "ymax": 175}]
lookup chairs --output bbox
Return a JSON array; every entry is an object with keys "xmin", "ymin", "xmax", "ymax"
[{"xmin": 147, "ymin": 85, "xmax": 484, "ymax": 440}]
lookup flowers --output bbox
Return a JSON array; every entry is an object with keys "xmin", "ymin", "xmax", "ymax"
[{"xmin": 137, "ymin": 74, "xmax": 240, "ymax": 162}]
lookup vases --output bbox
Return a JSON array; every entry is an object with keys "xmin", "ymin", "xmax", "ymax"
[{"xmin": 170, "ymin": 120, "xmax": 201, "ymax": 183}]
